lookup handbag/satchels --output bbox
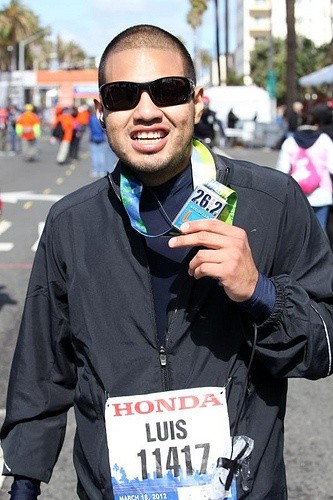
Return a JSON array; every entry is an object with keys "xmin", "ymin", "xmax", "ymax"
[{"xmin": 52, "ymin": 121, "xmax": 64, "ymax": 142}]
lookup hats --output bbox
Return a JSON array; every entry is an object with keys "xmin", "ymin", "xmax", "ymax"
[{"xmin": 24, "ymin": 104, "xmax": 33, "ymax": 111}]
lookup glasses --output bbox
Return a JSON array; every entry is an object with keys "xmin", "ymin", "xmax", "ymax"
[{"xmin": 100, "ymin": 76, "xmax": 193, "ymax": 111}]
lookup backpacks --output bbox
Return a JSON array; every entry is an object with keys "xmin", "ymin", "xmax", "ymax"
[{"xmin": 290, "ymin": 148, "xmax": 321, "ymax": 194}]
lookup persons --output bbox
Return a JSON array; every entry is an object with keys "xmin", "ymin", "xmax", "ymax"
[
  {"xmin": 0, "ymin": 94, "xmax": 333, "ymax": 247},
  {"xmin": 0, "ymin": 25, "xmax": 333, "ymax": 500}
]
